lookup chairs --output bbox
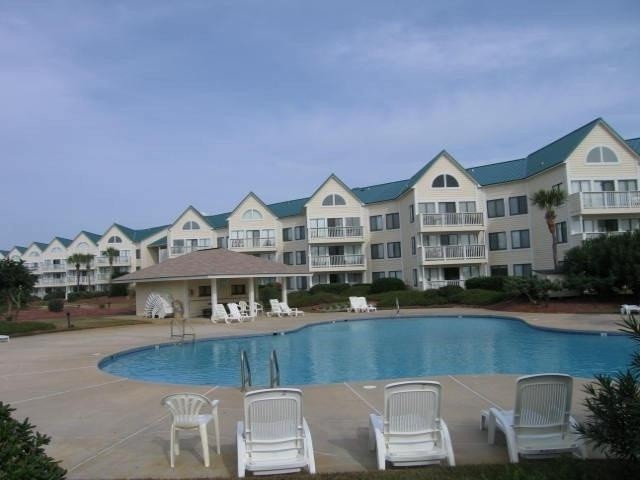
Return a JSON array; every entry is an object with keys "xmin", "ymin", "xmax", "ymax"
[
  {"xmin": 161, "ymin": 393, "xmax": 220, "ymax": 467},
  {"xmin": 211, "ymin": 299, "xmax": 304, "ymax": 324},
  {"xmin": 479, "ymin": 373, "xmax": 589, "ymax": 463},
  {"xmin": 369, "ymin": 381, "xmax": 455, "ymax": 471},
  {"xmin": 236, "ymin": 389, "xmax": 316, "ymax": 478},
  {"xmin": 348, "ymin": 296, "xmax": 377, "ymax": 313},
  {"xmin": 620, "ymin": 304, "xmax": 640, "ymax": 316}
]
